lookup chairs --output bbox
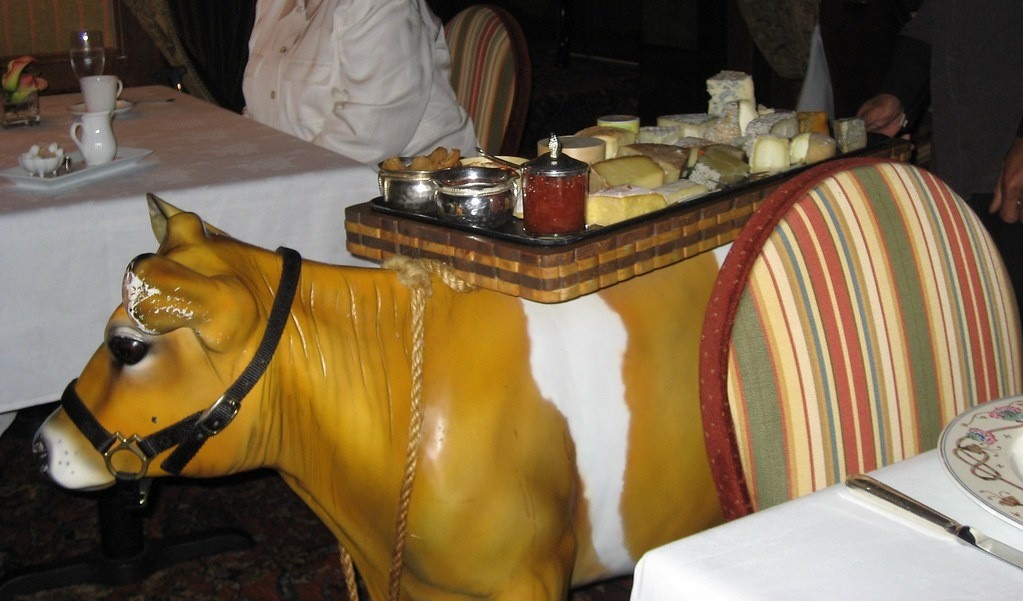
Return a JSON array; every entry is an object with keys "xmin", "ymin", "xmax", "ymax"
[
  {"xmin": 441, "ymin": 4, "xmax": 531, "ymax": 159},
  {"xmin": 698, "ymin": 156, "xmax": 1022, "ymax": 520}
]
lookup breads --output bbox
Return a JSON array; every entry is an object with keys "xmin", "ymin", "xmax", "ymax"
[{"xmin": 579, "ymin": 70, "xmax": 868, "ymax": 229}]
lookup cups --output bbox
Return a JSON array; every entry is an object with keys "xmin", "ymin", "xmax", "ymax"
[
  {"xmin": 596, "ymin": 115, "xmax": 640, "ymax": 145},
  {"xmin": 70, "ymin": 31, "xmax": 107, "ymax": 77}
]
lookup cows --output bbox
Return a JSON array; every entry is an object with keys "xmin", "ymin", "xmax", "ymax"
[{"xmin": 29, "ymin": 194, "xmax": 733, "ymax": 601}]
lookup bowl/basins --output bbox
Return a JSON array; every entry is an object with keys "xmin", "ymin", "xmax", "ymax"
[
  {"xmin": 429, "ymin": 165, "xmax": 520, "ymax": 232},
  {"xmin": 17, "ymin": 151, "xmax": 64, "ymax": 179},
  {"xmin": 375, "ymin": 156, "xmax": 464, "ymax": 215}
]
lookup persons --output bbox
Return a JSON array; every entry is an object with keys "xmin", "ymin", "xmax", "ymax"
[
  {"xmin": 243, "ymin": 0, "xmax": 483, "ymax": 165},
  {"xmin": 797, "ymin": 0, "xmax": 1023, "ymax": 343}
]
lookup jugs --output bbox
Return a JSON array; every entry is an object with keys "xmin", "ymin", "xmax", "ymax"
[
  {"xmin": 70, "ymin": 109, "xmax": 117, "ymax": 166},
  {"xmin": 79, "ymin": 75, "xmax": 124, "ymax": 113}
]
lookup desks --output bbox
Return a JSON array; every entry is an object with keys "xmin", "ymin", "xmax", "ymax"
[
  {"xmin": 630, "ymin": 448, "xmax": 1023, "ymax": 599},
  {"xmin": 0, "ymin": 86, "xmax": 382, "ymax": 446}
]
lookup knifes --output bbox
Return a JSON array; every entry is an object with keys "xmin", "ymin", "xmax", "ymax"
[{"xmin": 848, "ymin": 471, "xmax": 1022, "ymax": 570}]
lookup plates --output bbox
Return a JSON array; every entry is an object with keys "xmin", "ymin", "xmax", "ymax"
[
  {"xmin": 68, "ymin": 99, "xmax": 134, "ymax": 116},
  {"xmin": 2, "ymin": 143, "xmax": 154, "ymax": 186},
  {"xmin": 937, "ymin": 395, "xmax": 1023, "ymax": 523}
]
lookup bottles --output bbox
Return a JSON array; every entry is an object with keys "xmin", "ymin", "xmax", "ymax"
[{"xmin": 518, "ymin": 132, "xmax": 589, "ymax": 239}]
